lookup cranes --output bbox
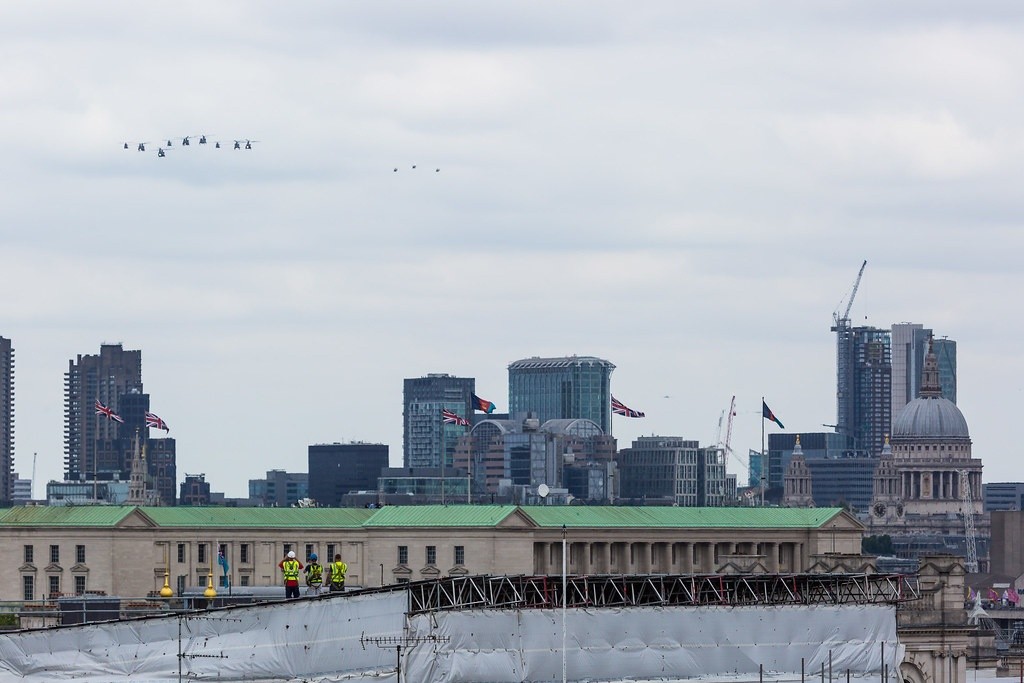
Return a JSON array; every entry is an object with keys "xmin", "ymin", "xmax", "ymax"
[
  {"xmin": 823, "ymin": 420, "xmax": 864, "ymax": 459},
  {"xmin": 720, "ymin": 441, "xmax": 769, "ymax": 490},
  {"xmin": 830, "ymin": 258, "xmax": 868, "ymax": 448},
  {"xmin": 711, "ymin": 392, "xmax": 735, "ymax": 468}
]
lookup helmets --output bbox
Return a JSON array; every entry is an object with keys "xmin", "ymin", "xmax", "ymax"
[
  {"xmin": 335, "ymin": 554, "xmax": 341, "ymax": 560},
  {"xmin": 287, "ymin": 551, "xmax": 296, "ymax": 558},
  {"xmin": 310, "ymin": 553, "xmax": 317, "ymax": 560}
]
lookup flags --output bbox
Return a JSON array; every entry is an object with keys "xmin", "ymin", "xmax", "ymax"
[
  {"xmin": 217, "ymin": 544, "xmax": 229, "ymax": 588},
  {"xmin": 95, "ymin": 399, "xmax": 123, "ymax": 423},
  {"xmin": 146, "ymin": 411, "xmax": 169, "ymax": 433},
  {"xmin": 611, "ymin": 396, "xmax": 645, "ymax": 418},
  {"xmin": 442, "ymin": 410, "xmax": 470, "ymax": 426},
  {"xmin": 471, "ymin": 392, "xmax": 495, "ymax": 413},
  {"xmin": 968, "ymin": 588, "xmax": 976, "ymax": 600},
  {"xmin": 763, "ymin": 402, "xmax": 784, "ymax": 428},
  {"xmin": 1008, "ymin": 589, "xmax": 1019, "ymax": 601},
  {"xmin": 988, "ymin": 589, "xmax": 998, "ymax": 603}
]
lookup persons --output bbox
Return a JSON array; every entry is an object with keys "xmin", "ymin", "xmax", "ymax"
[
  {"xmin": 278, "ymin": 551, "xmax": 303, "ymax": 598},
  {"xmin": 328, "ymin": 554, "xmax": 347, "ymax": 592},
  {"xmin": 304, "ymin": 554, "xmax": 323, "ymax": 595},
  {"xmin": 364, "ymin": 502, "xmax": 380, "ymax": 508}
]
